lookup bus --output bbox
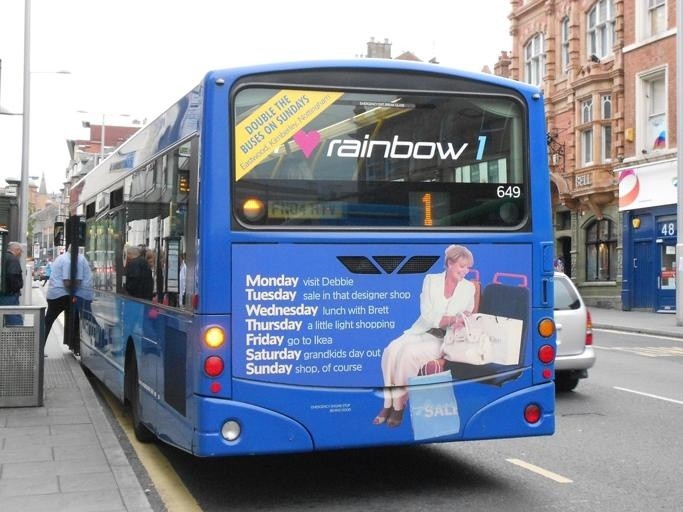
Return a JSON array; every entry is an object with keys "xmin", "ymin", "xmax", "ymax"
[{"xmin": 55, "ymin": 59, "xmax": 556, "ymax": 458}]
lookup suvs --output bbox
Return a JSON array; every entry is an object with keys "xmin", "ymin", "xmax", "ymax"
[{"xmin": 35, "ymin": 260, "xmax": 48, "ymax": 281}]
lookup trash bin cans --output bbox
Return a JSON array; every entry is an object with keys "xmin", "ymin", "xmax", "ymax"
[{"xmin": 0, "ymin": 305, "xmax": 46, "ymax": 407}]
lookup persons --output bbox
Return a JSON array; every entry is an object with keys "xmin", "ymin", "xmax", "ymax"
[
  {"xmin": 42, "ymin": 258, "xmax": 51, "ymax": 287},
  {"xmin": 76, "ymin": 254, "xmax": 109, "ymax": 355},
  {"xmin": 44, "ymin": 244, "xmax": 72, "ymax": 357},
  {"xmin": 372, "ymin": 245, "xmax": 477, "ymax": 427},
  {"xmin": 120, "ymin": 248, "xmax": 154, "ymax": 300},
  {"xmin": 0, "ymin": 241, "xmax": 24, "ymax": 326}
]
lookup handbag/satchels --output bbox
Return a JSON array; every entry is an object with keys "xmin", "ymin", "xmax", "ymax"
[
  {"xmin": 440, "ymin": 326, "xmax": 491, "ymax": 365},
  {"xmin": 408, "ymin": 370, "xmax": 460, "ymax": 441},
  {"xmin": 472, "ymin": 313, "xmax": 524, "ymax": 366}
]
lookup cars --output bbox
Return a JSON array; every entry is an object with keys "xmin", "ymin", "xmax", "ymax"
[{"xmin": 553, "ymin": 271, "xmax": 596, "ymax": 390}]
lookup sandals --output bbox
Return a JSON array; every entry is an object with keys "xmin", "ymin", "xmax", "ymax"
[
  {"xmin": 372, "ymin": 407, "xmax": 392, "ymax": 424},
  {"xmin": 387, "ymin": 401, "xmax": 406, "ymax": 428}
]
